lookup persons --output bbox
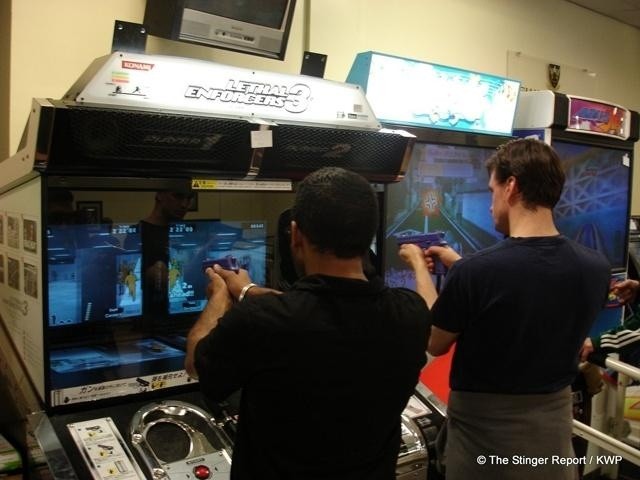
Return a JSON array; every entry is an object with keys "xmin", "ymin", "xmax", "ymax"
[
  {"xmin": 186, "ymin": 168, "xmax": 433, "ymax": 479},
  {"xmin": 398, "ymin": 137, "xmax": 611, "ymax": 480},
  {"xmin": 579, "ymin": 277, "xmax": 640, "ymax": 390}
]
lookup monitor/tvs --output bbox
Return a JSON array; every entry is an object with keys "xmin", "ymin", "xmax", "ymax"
[
  {"xmin": 44, "ymin": 221, "xmax": 145, "ymax": 327},
  {"xmin": 142, "ymin": 0, "xmax": 297, "ymax": 62},
  {"xmin": 387, "ymin": 141, "xmax": 509, "ymax": 292},
  {"xmin": 167, "ymin": 219, "xmax": 267, "ymax": 315},
  {"xmin": 551, "ymin": 139, "xmax": 632, "ymax": 270}
]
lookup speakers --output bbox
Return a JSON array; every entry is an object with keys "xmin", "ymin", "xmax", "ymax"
[
  {"xmin": 300, "ymin": 51, "xmax": 328, "ymax": 78},
  {"xmin": 112, "ymin": 20, "xmax": 148, "ymax": 53}
]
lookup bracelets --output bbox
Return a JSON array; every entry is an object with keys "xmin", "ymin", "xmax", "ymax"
[{"xmin": 238, "ymin": 282, "xmax": 258, "ymax": 303}]
